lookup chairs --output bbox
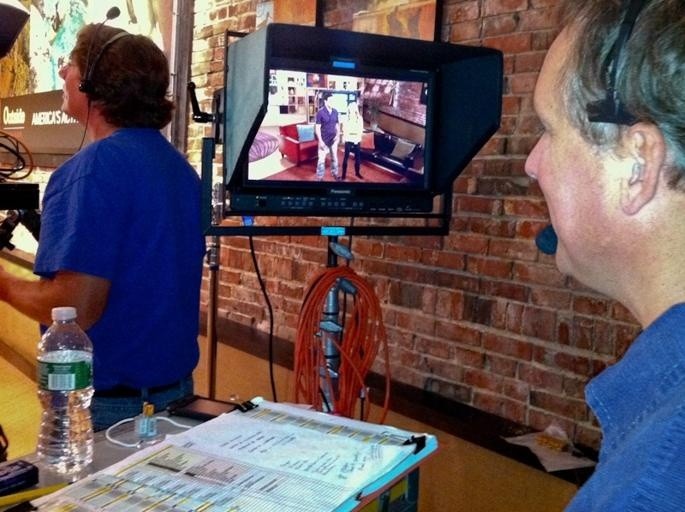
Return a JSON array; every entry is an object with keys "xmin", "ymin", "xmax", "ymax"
[{"xmin": 276, "ymin": 121, "xmax": 319, "ymax": 167}]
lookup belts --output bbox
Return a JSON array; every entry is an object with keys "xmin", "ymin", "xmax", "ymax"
[{"xmin": 93, "ymin": 381, "xmax": 180, "ymax": 398}]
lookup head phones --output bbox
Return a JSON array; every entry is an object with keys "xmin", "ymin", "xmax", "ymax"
[{"xmin": 79, "ymin": 30, "xmax": 133, "ymax": 97}]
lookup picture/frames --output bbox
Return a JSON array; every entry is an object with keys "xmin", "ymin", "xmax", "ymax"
[
  {"xmin": 280, "ymin": 74, "xmax": 329, "ymax": 123},
  {"xmin": 0, "ymin": 0, "xmax": 195, "ymax": 170}
]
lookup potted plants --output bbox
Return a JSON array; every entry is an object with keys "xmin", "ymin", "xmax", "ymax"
[{"xmin": 366, "ymin": 98, "xmax": 382, "ymax": 129}]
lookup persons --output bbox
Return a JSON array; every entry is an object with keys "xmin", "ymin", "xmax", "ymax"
[
  {"xmin": 0, "ymin": 22, "xmax": 207, "ymax": 434},
  {"xmin": 522, "ymin": 0, "xmax": 685, "ymax": 511},
  {"xmin": 342, "ymin": 101, "xmax": 364, "ymax": 181},
  {"xmin": 314, "ymin": 93, "xmax": 342, "ymax": 182}
]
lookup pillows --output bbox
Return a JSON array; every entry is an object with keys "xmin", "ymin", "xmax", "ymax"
[
  {"xmin": 360, "ymin": 132, "xmax": 375, "ymax": 149},
  {"xmin": 296, "ymin": 124, "xmax": 315, "ymax": 142},
  {"xmin": 390, "ymin": 138, "xmax": 416, "ymax": 160}
]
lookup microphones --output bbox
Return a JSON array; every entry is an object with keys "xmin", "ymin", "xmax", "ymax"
[
  {"xmin": 536, "ymin": 224, "xmax": 560, "ymax": 253},
  {"xmin": 79, "ymin": 5, "xmax": 120, "ymax": 92}
]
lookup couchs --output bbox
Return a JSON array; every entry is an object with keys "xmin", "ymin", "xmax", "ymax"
[{"xmin": 359, "ymin": 129, "xmax": 424, "ymax": 177}]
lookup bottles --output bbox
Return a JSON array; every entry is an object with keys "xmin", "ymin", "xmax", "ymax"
[{"xmin": 34, "ymin": 306, "xmax": 96, "ymax": 477}]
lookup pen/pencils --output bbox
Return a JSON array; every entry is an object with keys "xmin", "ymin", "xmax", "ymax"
[{"xmin": 0, "ymin": 481, "xmax": 75, "ymax": 508}]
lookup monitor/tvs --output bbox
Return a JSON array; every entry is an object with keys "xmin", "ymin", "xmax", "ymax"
[{"xmin": 242, "ymin": 58, "xmax": 434, "ymax": 194}]
